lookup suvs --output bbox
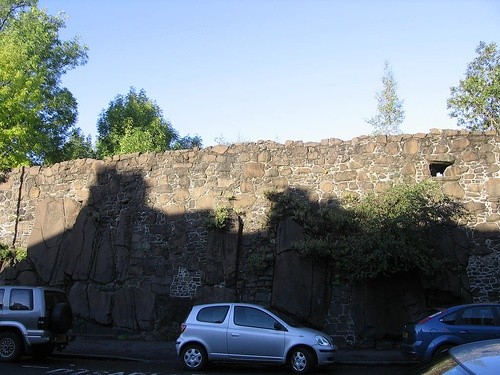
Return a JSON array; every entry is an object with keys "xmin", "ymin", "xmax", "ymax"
[{"xmin": 0, "ymin": 286, "xmax": 73, "ymax": 363}]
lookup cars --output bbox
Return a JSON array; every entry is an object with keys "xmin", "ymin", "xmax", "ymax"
[
  {"xmin": 400, "ymin": 302, "xmax": 500, "ymax": 362},
  {"xmin": 396, "ymin": 339, "xmax": 500, "ymax": 375},
  {"xmin": 176, "ymin": 303, "xmax": 337, "ymax": 375}
]
